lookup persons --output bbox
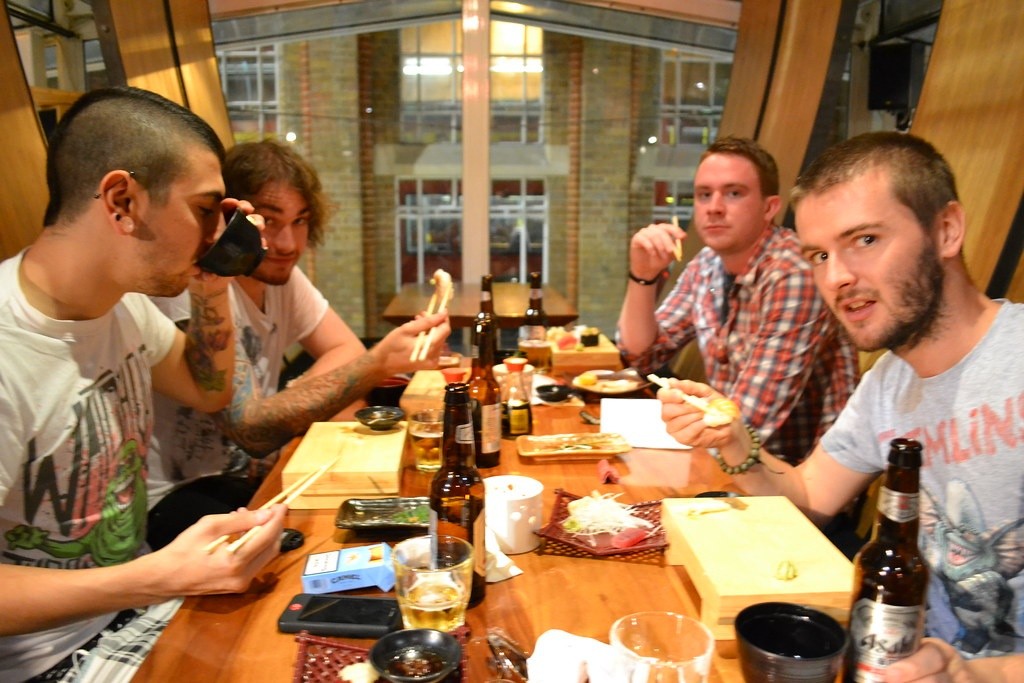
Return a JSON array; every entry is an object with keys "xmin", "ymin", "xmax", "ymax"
[
  {"xmin": 130, "ymin": 138, "xmax": 451, "ymax": 550},
  {"xmin": 657, "ymin": 131, "xmax": 1023, "ymax": 683},
  {"xmin": 615, "ymin": 137, "xmax": 865, "ymax": 546},
  {"xmin": 0, "ymin": 87, "xmax": 290, "ymax": 683}
]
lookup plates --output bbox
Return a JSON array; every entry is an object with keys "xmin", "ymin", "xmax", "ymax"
[
  {"xmin": 571, "ymin": 366, "xmax": 654, "ymax": 398},
  {"xmin": 338, "ymin": 498, "xmax": 430, "ymax": 531},
  {"xmin": 516, "ymin": 431, "xmax": 631, "ymax": 462}
]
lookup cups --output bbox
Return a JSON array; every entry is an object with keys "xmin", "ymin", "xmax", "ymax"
[
  {"xmin": 389, "ymin": 535, "xmax": 476, "ymax": 632},
  {"xmin": 518, "ymin": 338, "xmax": 552, "ymax": 375},
  {"xmin": 733, "ymin": 601, "xmax": 851, "ymax": 682},
  {"xmin": 408, "ymin": 406, "xmax": 443, "ymax": 471},
  {"xmin": 478, "ymin": 475, "xmax": 544, "ymax": 556},
  {"xmin": 608, "ymin": 610, "xmax": 715, "ymax": 683}
]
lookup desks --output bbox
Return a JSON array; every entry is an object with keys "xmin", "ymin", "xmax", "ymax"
[{"xmin": 383, "ymin": 283, "xmax": 580, "ymax": 354}]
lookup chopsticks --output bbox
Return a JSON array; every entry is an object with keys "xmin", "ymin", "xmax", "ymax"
[
  {"xmin": 409, "ymin": 281, "xmax": 452, "ymax": 364},
  {"xmin": 666, "ymin": 215, "xmax": 683, "ymax": 264},
  {"xmin": 645, "ymin": 373, "xmax": 733, "ymax": 425},
  {"xmin": 204, "ymin": 455, "xmax": 341, "ymax": 556}
]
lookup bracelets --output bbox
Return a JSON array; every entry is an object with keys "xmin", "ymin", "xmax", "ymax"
[
  {"xmin": 629, "ymin": 272, "xmax": 660, "ymax": 287},
  {"xmin": 715, "ymin": 424, "xmax": 760, "ymax": 474}
]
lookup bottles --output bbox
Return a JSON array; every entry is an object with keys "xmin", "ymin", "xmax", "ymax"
[
  {"xmin": 518, "ymin": 271, "xmax": 548, "ymax": 340},
  {"xmin": 465, "ymin": 318, "xmax": 501, "ymax": 469},
  {"xmin": 841, "ymin": 437, "xmax": 931, "ymax": 683},
  {"xmin": 503, "ymin": 357, "xmax": 533, "ymax": 440},
  {"xmin": 477, "ymin": 274, "xmax": 499, "ymax": 367},
  {"xmin": 428, "ymin": 381, "xmax": 485, "ymax": 610},
  {"xmin": 440, "ymin": 366, "xmax": 467, "ymax": 386}
]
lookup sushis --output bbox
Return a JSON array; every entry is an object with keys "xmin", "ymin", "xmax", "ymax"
[
  {"xmin": 558, "ymin": 326, "xmax": 600, "ymax": 350},
  {"xmin": 704, "ymin": 397, "xmax": 741, "ymax": 428},
  {"xmin": 433, "ymin": 269, "xmax": 454, "ymax": 299}
]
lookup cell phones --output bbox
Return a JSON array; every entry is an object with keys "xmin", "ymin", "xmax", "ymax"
[{"xmin": 278, "ymin": 593, "xmax": 402, "ymax": 639}]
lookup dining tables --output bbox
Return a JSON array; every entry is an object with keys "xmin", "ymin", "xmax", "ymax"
[{"xmin": 129, "ymin": 358, "xmax": 858, "ymax": 683}]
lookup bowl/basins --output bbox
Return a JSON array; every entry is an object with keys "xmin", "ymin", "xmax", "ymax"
[
  {"xmin": 536, "ymin": 383, "xmax": 570, "ymax": 402},
  {"xmin": 354, "ymin": 405, "xmax": 404, "ymax": 431},
  {"xmin": 363, "ymin": 375, "xmax": 409, "ymax": 406},
  {"xmin": 369, "ymin": 626, "xmax": 461, "ymax": 683}
]
